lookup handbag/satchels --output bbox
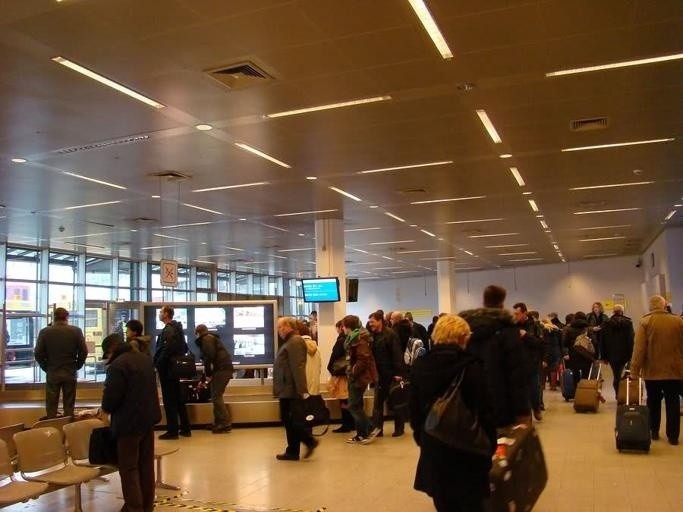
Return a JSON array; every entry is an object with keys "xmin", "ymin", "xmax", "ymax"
[
  {"xmin": 290, "ymin": 395, "xmax": 330, "ymax": 428},
  {"xmin": 425, "ymin": 383, "xmax": 492, "ymax": 457},
  {"xmin": 332, "ymin": 356, "xmax": 350, "ymax": 374},
  {"xmin": 389, "ymin": 380, "xmax": 412, "ymax": 411},
  {"xmin": 196, "ymin": 381, "xmax": 210, "ymax": 401},
  {"xmin": 177, "ymin": 350, "xmax": 196, "ymax": 378},
  {"xmin": 90, "ymin": 427, "xmax": 117, "ymax": 464},
  {"xmin": 573, "ymin": 335, "xmax": 596, "ymax": 359}
]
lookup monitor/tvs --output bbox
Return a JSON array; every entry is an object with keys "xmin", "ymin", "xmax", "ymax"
[{"xmin": 301, "ymin": 277, "xmax": 340, "ymax": 302}]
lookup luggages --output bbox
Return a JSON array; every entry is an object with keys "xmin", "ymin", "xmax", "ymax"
[
  {"xmin": 615, "ymin": 360, "xmax": 651, "ymax": 454},
  {"xmin": 561, "ymin": 355, "xmax": 603, "ymax": 413},
  {"xmin": 489, "ymin": 420, "xmax": 547, "ymax": 512}
]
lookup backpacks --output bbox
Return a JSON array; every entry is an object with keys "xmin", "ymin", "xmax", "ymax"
[{"xmin": 397, "ymin": 328, "xmax": 427, "ymax": 367}]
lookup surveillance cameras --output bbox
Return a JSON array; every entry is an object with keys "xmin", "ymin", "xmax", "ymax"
[{"xmin": 635, "ymin": 260, "xmax": 641, "ymax": 267}]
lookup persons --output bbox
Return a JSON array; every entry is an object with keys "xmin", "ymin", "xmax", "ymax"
[
  {"xmin": 36, "ymin": 309, "xmax": 88, "ymax": 418},
  {"xmin": 409, "ymin": 313, "xmax": 497, "ymax": 511},
  {"xmin": 629, "ymin": 293, "xmax": 682, "ymax": 446},
  {"xmin": 326, "ymin": 310, "xmax": 448, "ymax": 445},
  {"xmin": 273, "ymin": 311, "xmax": 322, "ymax": 461},
  {"xmin": 542, "ymin": 294, "xmax": 634, "ymax": 402},
  {"xmin": 456, "ymin": 285, "xmax": 550, "ymax": 441},
  {"xmin": 92, "ymin": 307, "xmax": 235, "ymax": 511}
]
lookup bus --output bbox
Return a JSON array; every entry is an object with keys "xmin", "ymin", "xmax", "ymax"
[{"xmin": 47, "ymin": 294, "xmax": 132, "ymax": 372}]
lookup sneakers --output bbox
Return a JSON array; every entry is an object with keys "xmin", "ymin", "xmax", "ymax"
[
  {"xmin": 332, "ymin": 425, "xmax": 405, "ymax": 445},
  {"xmin": 534, "ymin": 408, "xmax": 542, "ymax": 420},
  {"xmin": 276, "ymin": 452, "xmax": 299, "ymax": 460},
  {"xmin": 213, "ymin": 424, "xmax": 231, "ymax": 432},
  {"xmin": 180, "ymin": 431, "xmax": 190, "ymax": 436},
  {"xmin": 159, "ymin": 432, "xmax": 179, "ymax": 440}
]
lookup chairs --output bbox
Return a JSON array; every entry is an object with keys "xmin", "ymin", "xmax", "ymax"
[{"xmin": 0, "ymin": 407, "xmax": 180, "ymax": 512}]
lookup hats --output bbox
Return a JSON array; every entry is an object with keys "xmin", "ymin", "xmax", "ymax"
[{"xmin": 102, "ymin": 334, "xmax": 121, "ymax": 359}]
